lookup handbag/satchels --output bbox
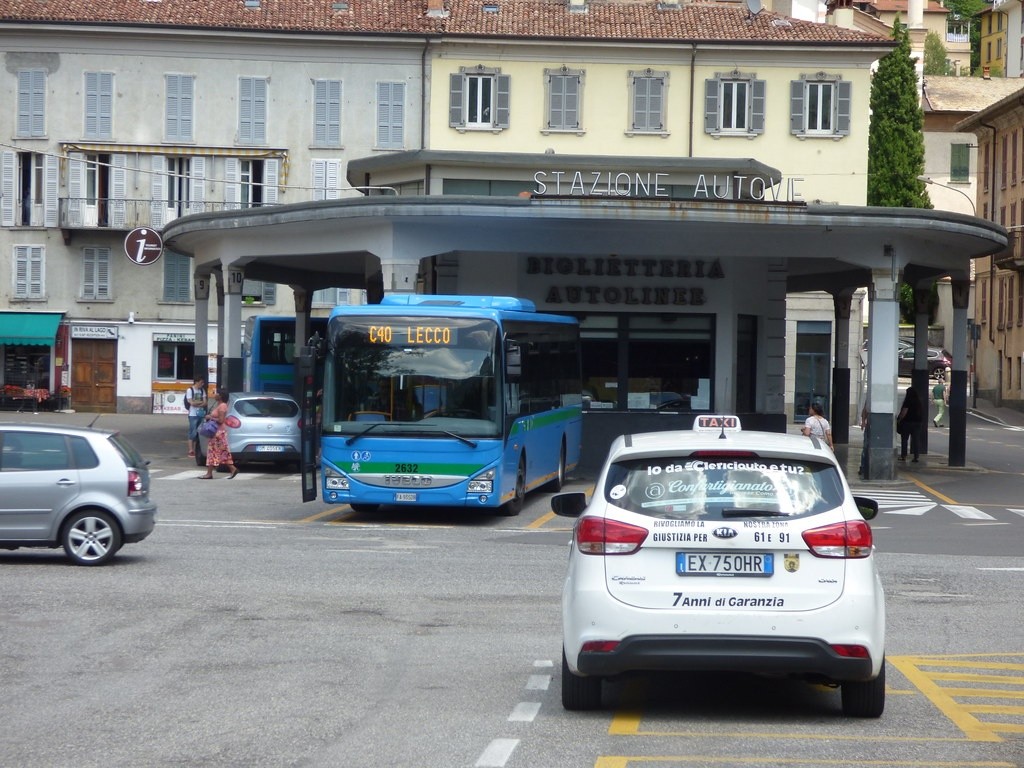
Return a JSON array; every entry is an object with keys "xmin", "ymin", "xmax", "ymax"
[
  {"xmin": 896, "ymin": 412, "xmax": 903, "ymax": 433},
  {"xmin": 199, "ymin": 418, "xmax": 218, "ymax": 438}
]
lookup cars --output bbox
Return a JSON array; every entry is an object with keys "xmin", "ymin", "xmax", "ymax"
[
  {"xmin": 0, "ymin": 421, "xmax": 159, "ymax": 565},
  {"xmin": 897, "ymin": 348, "xmax": 952, "ymax": 379},
  {"xmin": 858, "ymin": 335, "xmax": 937, "ymax": 370},
  {"xmin": 549, "ymin": 413, "xmax": 887, "ymax": 718},
  {"xmin": 193, "ymin": 390, "xmax": 306, "ymax": 475}
]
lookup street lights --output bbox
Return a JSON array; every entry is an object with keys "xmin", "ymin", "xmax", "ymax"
[{"xmin": 916, "ymin": 170, "xmax": 977, "ymax": 218}]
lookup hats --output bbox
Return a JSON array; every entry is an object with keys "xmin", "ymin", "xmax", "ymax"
[
  {"xmin": 317, "ymin": 389, "xmax": 323, "ymax": 397},
  {"xmin": 938, "ymin": 378, "xmax": 945, "ymax": 383}
]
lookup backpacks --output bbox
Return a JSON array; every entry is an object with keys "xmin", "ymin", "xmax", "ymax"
[{"xmin": 184, "ymin": 387, "xmax": 203, "ymax": 410}]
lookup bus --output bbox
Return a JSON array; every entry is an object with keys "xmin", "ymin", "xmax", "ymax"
[
  {"xmin": 244, "ymin": 312, "xmax": 332, "ymax": 409},
  {"xmin": 298, "ymin": 289, "xmax": 586, "ymax": 523}
]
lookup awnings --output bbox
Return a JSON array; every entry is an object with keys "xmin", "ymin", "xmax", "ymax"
[{"xmin": 0, "ymin": 313, "xmax": 65, "ymax": 346}]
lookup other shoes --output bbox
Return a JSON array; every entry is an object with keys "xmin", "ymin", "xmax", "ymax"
[
  {"xmin": 939, "ymin": 423, "xmax": 944, "ymax": 427},
  {"xmin": 858, "ymin": 471, "xmax": 865, "ymax": 475},
  {"xmin": 933, "ymin": 419, "xmax": 938, "ymax": 427},
  {"xmin": 227, "ymin": 468, "xmax": 239, "ymax": 479},
  {"xmin": 199, "ymin": 474, "xmax": 213, "ymax": 479},
  {"xmin": 898, "ymin": 456, "xmax": 907, "ymax": 461},
  {"xmin": 912, "ymin": 457, "xmax": 920, "ymax": 462},
  {"xmin": 188, "ymin": 451, "xmax": 195, "ymax": 458}
]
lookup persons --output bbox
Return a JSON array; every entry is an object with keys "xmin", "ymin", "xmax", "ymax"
[
  {"xmin": 367, "ymin": 377, "xmax": 411, "ymax": 421},
  {"xmin": 184, "ymin": 373, "xmax": 239, "ymax": 480},
  {"xmin": 858, "ymin": 400, "xmax": 869, "ymax": 475},
  {"xmin": 932, "ymin": 378, "xmax": 949, "ymax": 428},
  {"xmin": 315, "ymin": 387, "xmax": 322, "ymax": 460},
  {"xmin": 801, "ymin": 402, "xmax": 834, "ymax": 452},
  {"xmin": 896, "ymin": 387, "xmax": 923, "ymax": 463},
  {"xmin": 16, "ymin": 362, "xmax": 40, "ymax": 415}
]
